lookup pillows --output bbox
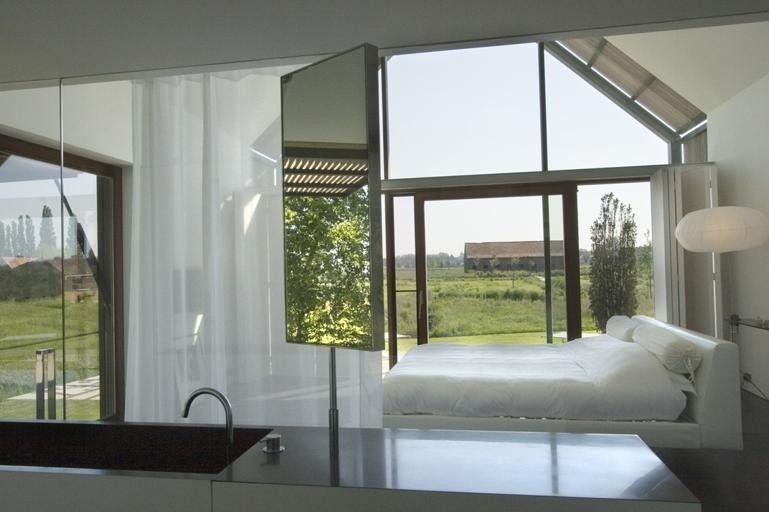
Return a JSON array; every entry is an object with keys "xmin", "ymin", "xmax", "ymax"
[{"xmin": 554, "ymin": 314, "xmax": 701, "ymax": 385}]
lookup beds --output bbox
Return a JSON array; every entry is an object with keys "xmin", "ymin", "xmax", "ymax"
[{"xmin": 382, "ymin": 313, "xmax": 745, "ymax": 452}]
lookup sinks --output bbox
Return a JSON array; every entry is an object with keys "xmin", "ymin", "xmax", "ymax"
[{"xmin": 0, "ymin": 418, "xmax": 275, "ymax": 481}]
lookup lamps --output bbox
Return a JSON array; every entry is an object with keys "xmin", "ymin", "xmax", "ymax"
[{"xmin": 674, "ymin": 206, "xmax": 769, "ymax": 400}]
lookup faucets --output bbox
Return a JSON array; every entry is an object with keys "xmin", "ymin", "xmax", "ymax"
[
  {"xmin": 326, "ymin": 345, "xmax": 337, "ymax": 429},
  {"xmin": 180, "ymin": 388, "xmax": 236, "ymax": 451}
]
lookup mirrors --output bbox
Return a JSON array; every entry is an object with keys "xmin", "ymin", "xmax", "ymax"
[{"xmin": 280, "ymin": 42, "xmax": 385, "ymax": 352}]
